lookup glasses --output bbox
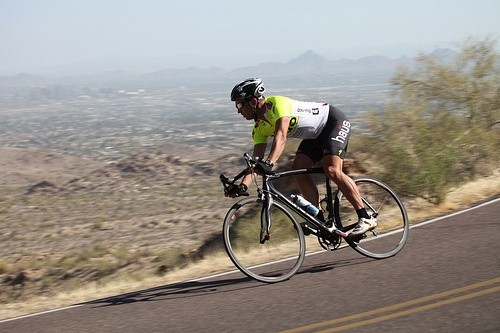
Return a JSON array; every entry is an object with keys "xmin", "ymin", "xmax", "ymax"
[{"xmin": 236, "ymin": 100, "xmax": 250, "ymax": 109}]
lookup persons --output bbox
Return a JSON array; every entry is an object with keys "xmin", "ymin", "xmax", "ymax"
[{"xmin": 224, "ymin": 77, "xmax": 376, "ymax": 238}]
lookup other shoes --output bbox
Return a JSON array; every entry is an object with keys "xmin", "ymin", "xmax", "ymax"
[
  {"xmin": 294, "ymin": 221, "xmax": 327, "ymax": 232},
  {"xmin": 348, "ymin": 215, "xmax": 377, "ymax": 237}
]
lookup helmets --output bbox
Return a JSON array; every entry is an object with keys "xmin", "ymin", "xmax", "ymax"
[{"xmin": 230, "ymin": 78, "xmax": 266, "ymax": 101}]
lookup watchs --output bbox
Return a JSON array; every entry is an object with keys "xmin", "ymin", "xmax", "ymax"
[{"xmin": 266, "ymin": 159, "xmax": 274, "ymax": 167}]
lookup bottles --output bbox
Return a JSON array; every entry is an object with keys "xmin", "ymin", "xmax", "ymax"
[{"xmin": 290, "ymin": 193, "xmax": 320, "ymax": 217}]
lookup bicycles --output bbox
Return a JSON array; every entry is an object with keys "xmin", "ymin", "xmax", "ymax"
[{"xmin": 217, "ymin": 152, "xmax": 410, "ymax": 284}]
